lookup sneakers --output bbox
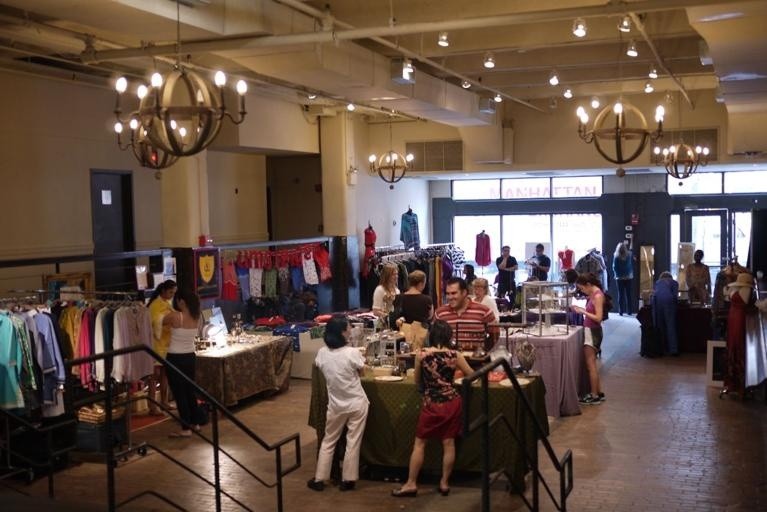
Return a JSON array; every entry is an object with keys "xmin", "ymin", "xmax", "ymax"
[
  {"xmin": 578, "ymin": 393, "xmax": 601, "ymax": 405},
  {"xmin": 307, "ymin": 477, "xmax": 324, "ymax": 491},
  {"xmin": 340, "ymin": 480, "xmax": 356, "ymax": 491},
  {"xmin": 161, "ymin": 405, "xmax": 176, "ymax": 410},
  {"xmin": 149, "ymin": 408, "xmax": 165, "ymax": 417},
  {"xmin": 598, "ymin": 392, "xmax": 605, "ymax": 401}
]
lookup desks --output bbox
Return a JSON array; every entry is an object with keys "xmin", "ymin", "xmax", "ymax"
[
  {"xmin": 187, "ymin": 334, "xmax": 292, "ymax": 417},
  {"xmin": 636, "ymin": 304, "xmax": 715, "ymax": 359}
]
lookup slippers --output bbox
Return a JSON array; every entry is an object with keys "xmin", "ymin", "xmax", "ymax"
[
  {"xmin": 168, "ymin": 430, "xmax": 193, "ymax": 438},
  {"xmin": 392, "ymin": 487, "xmax": 417, "ymax": 497},
  {"xmin": 439, "ymin": 486, "xmax": 450, "ymax": 496}
]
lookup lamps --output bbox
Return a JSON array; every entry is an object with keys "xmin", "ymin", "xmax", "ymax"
[
  {"xmin": 367, "ymin": 109, "xmax": 418, "ymax": 190},
  {"xmin": 110, "ymin": 0, "xmax": 249, "ymax": 182}
]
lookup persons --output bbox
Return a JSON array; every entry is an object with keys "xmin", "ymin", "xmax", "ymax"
[
  {"xmin": 397, "ymin": 270, "xmax": 435, "ymax": 347},
  {"xmin": 564, "ymin": 269, "xmax": 584, "ymax": 326},
  {"xmin": 371, "ymin": 262, "xmax": 400, "ymax": 333},
  {"xmin": 570, "ymin": 272, "xmax": 607, "ymax": 405},
  {"xmin": 399, "ymin": 208, "xmax": 420, "ymax": 251},
  {"xmin": 649, "ymin": 272, "xmax": 680, "ymax": 359},
  {"xmin": 144, "ymin": 280, "xmax": 180, "ymax": 416},
  {"xmin": 152, "ymin": 290, "xmax": 202, "ymax": 437},
  {"xmin": 461, "ymin": 263, "xmax": 476, "ymax": 287},
  {"xmin": 523, "ymin": 244, "xmax": 551, "ymax": 282},
  {"xmin": 474, "ymin": 230, "xmax": 492, "ymax": 267},
  {"xmin": 442, "ymin": 254, "xmax": 453, "ymax": 282},
  {"xmin": 306, "ymin": 315, "xmax": 368, "ymax": 492},
  {"xmin": 362, "ymin": 226, "xmax": 377, "ymax": 280},
  {"xmin": 684, "ymin": 250, "xmax": 711, "ymax": 302},
  {"xmin": 722, "ymin": 274, "xmax": 753, "ymax": 396},
  {"xmin": 390, "ymin": 318, "xmax": 473, "ymax": 497},
  {"xmin": 611, "ymin": 241, "xmax": 636, "ymax": 317},
  {"xmin": 469, "ymin": 278, "xmax": 499, "ymax": 325},
  {"xmin": 431, "ymin": 276, "xmax": 499, "ymax": 354},
  {"xmin": 557, "ymin": 246, "xmax": 575, "ymax": 290},
  {"xmin": 495, "ymin": 246, "xmax": 518, "ymax": 306}
]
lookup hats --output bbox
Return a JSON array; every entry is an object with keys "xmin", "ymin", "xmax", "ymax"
[{"xmin": 728, "ymin": 273, "xmax": 753, "ymax": 288}]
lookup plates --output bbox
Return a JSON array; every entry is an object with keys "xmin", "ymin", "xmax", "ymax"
[
  {"xmin": 455, "ymin": 377, "xmax": 478, "ymax": 384},
  {"xmin": 499, "ymin": 378, "xmax": 531, "ymax": 387},
  {"xmin": 374, "ymin": 376, "xmax": 404, "ymax": 382}
]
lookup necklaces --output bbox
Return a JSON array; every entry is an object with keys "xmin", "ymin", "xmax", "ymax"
[{"xmin": 516, "ymin": 345, "xmax": 536, "ymax": 364}]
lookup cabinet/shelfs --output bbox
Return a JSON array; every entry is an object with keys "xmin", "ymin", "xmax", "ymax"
[{"xmin": 520, "ymin": 280, "xmax": 570, "ymax": 336}]
